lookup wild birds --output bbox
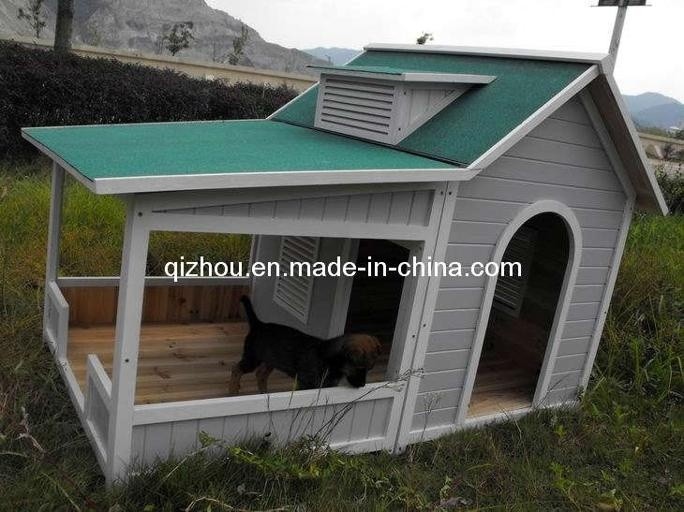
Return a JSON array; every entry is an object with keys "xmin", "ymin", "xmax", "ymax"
[{"xmin": 417, "ymin": 33, "xmax": 430, "ymax": 44}]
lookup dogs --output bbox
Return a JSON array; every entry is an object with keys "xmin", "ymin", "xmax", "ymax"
[{"xmin": 228, "ymin": 295, "xmax": 384, "ymax": 398}]
